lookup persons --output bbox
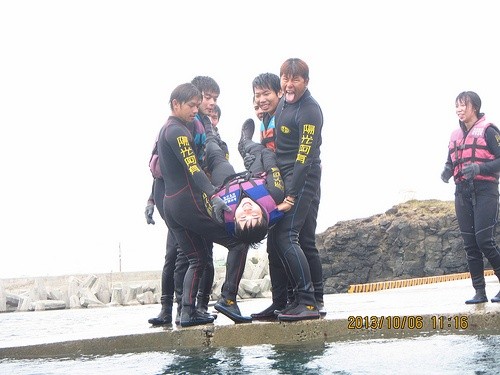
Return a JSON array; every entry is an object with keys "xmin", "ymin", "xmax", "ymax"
[
  {"xmin": 136, "ymin": 56, "xmax": 327, "ymax": 327},
  {"xmin": 441, "ymin": 92, "xmax": 500, "ymax": 304}
]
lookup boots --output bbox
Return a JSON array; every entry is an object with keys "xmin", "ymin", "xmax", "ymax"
[
  {"xmin": 238, "ymin": 118, "xmax": 255, "ymax": 150},
  {"xmin": 199, "ymin": 115, "xmax": 213, "ymax": 133},
  {"xmin": 197, "ymin": 291, "xmax": 218, "ymax": 320},
  {"xmin": 148, "ymin": 294, "xmax": 173, "ymax": 327}
]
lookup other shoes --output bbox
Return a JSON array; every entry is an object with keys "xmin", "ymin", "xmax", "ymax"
[
  {"xmin": 274, "ymin": 304, "xmax": 320, "ymax": 320},
  {"xmin": 251, "ymin": 304, "xmax": 284, "ymax": 320},
  {"xmin": 316, "ymin": 302, "xmax": 324, "ymax": 316}
]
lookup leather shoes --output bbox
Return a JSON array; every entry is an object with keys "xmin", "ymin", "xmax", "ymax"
[
  {"xmin": 214, "ymin": 293, "xmax": 253, "ymax": 322},
  {"xmin": 175, "ymin": 301, "xmax": 182, "ymax": 325},
  {"xmin": 491, "ymin": 292, "xmax": 500, "ymax": 302},
  {"xmin": 465, "ymin": 290, "xmax": 488, "ymax": 304},
  {"xmin": 181, "ymin": 305, "xmax": 214, "ymax": 326}
]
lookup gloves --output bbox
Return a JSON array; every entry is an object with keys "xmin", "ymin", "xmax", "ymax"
[
  {"xmin": 441, "ymin": 165, "xmax": 452, "ymax": 182},
  {"xmin": 145, "ymin": 202, "xmax": 155, "ymax": 225},
  {"xmin": 211, "ymin": 195, "xmax": 232, "ymax": 224},
  {"xmin": 243, "ymin": 152, "xmax": 255, "ymax": 170},
  {"xmin": 461, "ymin": 164, "xmax": 480, "ymax": 180}
]
